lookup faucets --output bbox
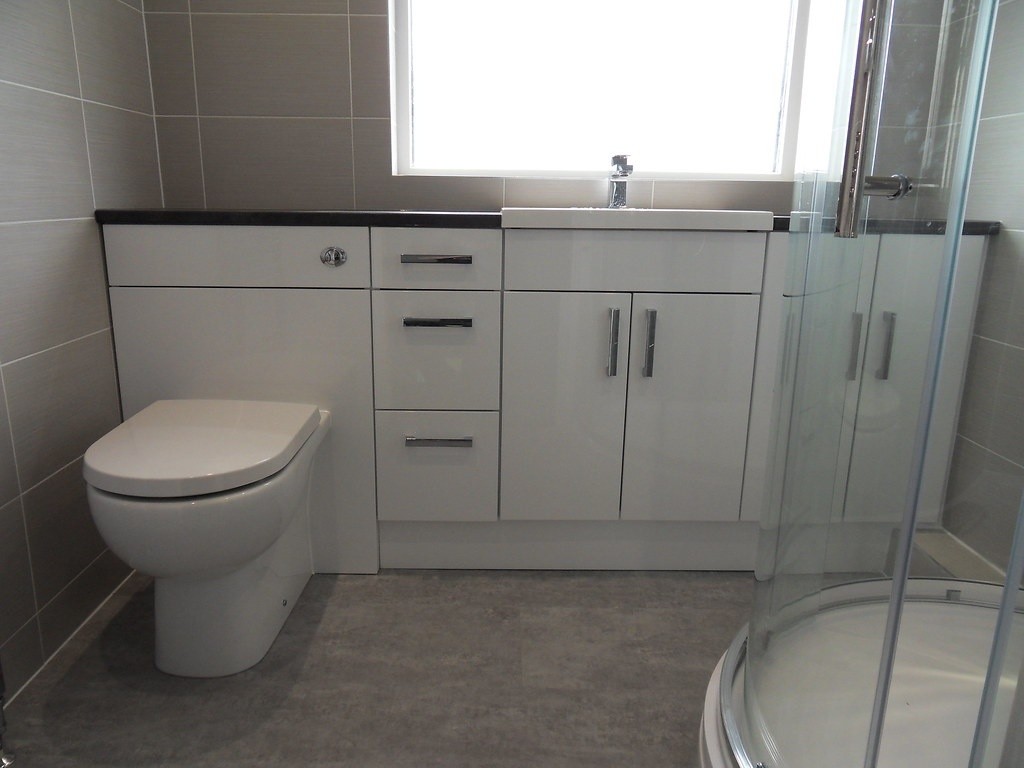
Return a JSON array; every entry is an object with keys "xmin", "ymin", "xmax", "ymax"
[{"xmin": 607, "ymin": 154, "xmax": 634, "ymax": 209}]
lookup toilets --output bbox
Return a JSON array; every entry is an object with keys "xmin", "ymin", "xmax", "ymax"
[{"xmin": 80, "ymin": 394, "xmax": 332, "ymax": 679}]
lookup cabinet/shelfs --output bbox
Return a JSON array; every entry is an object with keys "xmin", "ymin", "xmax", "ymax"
[{"xmin": 102, "ymin": 224, "xmax": 987, "ymax": 580}]
